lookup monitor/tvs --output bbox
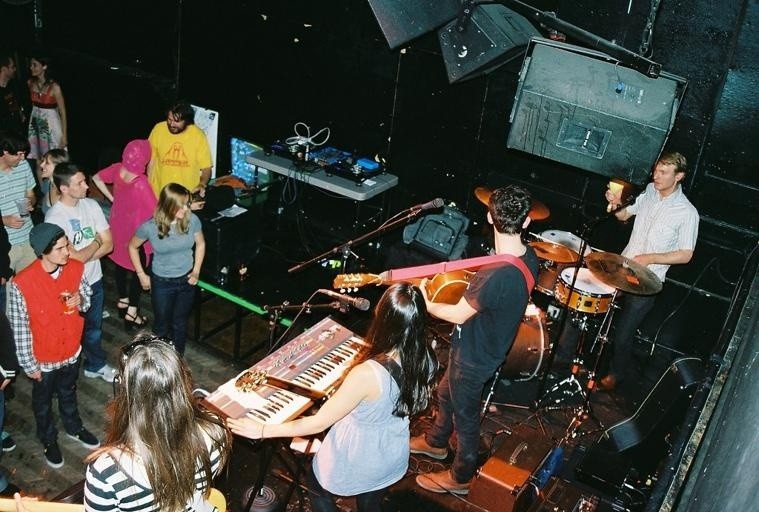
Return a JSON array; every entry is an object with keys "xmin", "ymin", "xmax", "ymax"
[{"xmin": 505, "ymin": 36, "xmax": 689, "ymax": 187}]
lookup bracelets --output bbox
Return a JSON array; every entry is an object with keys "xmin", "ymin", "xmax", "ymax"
[{"xmin": 94, "ymin": 238, "xmax": 104, "ymax": 246}]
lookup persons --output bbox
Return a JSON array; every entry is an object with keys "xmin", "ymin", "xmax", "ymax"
[
  {"xmin": 128, "ymin": 184, "xmax": 206, "ymax": 353},
  {"xmin": 37, "ymin": 153, "xmax": 75, "ymax": 215},
  {"xmin": 409, "ymin": 185, "xmax": 539, "ymax": 493},
  {"xmin": 147, "ymin": 103, "xmax": 212, "ymax": 195},
  {"xmin": 11, "ymin": 224, "xmax": 101, "ymax": 469},
  {"xmin": 24, "ymin": 53, "xmax": 66, "ymax": 163},
  {"xmin": 596, "ymin": 153, "xmax": 700, "ymax": 386},
  {"xmin": 0, "ymin": 137, "xmax": 39, "ymax": 269},
  {"xmin": 223, "ymin": 281, "xmax": 437, "ymax": 511},
  {"xmin": 95, "ymin": 143, "xmax": 158, "ymax": 331},
  {"xmin": 44, "ymin": 164, "xmax": 125, "ymax": 385},
  {"xmin": 0, "ymin": 312, "xmax": 24, "ymax": 500},
  {"xmin": 12, "ymin": 335, "xmax": 232, "ymax": 512},
  {"xmin": 1, "ymin": 56, "xmax": 29, "ymax": 148}
]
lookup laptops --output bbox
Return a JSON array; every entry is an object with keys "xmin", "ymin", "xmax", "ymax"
[{"xmin": 207, "ymin": 138, "xmax": 270, "ymax": 207}]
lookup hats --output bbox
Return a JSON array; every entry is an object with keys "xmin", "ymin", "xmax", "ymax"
[{"xmin": 29, "ymin": 223, "xmax": 64, "ymax": 258}]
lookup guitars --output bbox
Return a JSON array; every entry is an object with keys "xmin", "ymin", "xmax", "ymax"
[
  {"xmin": 234, "ymin": 371, "xmax": 341, "ymax": 438},
  {"xmin": 0, "ymin": 485, "xmax": 226, "ymax": 512},
  {"xmin": 333, "ymin": 268, "xmax": 477, "ymax": 320}
]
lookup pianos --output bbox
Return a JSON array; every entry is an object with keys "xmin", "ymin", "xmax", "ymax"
[{"xmin": 203, "ymin": 316, "xmax": 373, "ymax": 450}]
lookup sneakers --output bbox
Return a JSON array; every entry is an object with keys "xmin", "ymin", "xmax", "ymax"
[
  {"xmin": 84, "ymin": 365, "xmax": 121, "ymax": 383},
  {"xmin": 0, "ymin": 482, "xmax": 21, "ymax": 498},
  {"xmin": 44, "ymin": 441, "xmax": 63, "ymax": 468},
  {"xmin": 67, "ymin": 427, "xmax": 100, "ymax": 448},
  {"xmin": 1, "ymin": 431, "xmax": 16, "ymax": 451}
]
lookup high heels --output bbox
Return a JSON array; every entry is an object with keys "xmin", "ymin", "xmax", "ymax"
[
  {"xmin": 117, "ymin": 301, "xmax": 129, "ymax": 318},
  {"xmin": 125, "ymin": 313, "xmax": 147, "ymax": 330}
]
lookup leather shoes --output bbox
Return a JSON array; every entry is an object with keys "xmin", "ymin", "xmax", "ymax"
[
  {"xmin": 572, "ymin": 360, "xmax": 597, "ymax": 374},
  {"xmin": 588, "ymin": 374, "xmax": 618, "ymax": 392},
  {"xmin": 417, "ymin": 470, "xmax": 471, "ymax": 495},
  {"xmin": 410, "ymin": 434, "xmax": 448, "ymax": 459}
]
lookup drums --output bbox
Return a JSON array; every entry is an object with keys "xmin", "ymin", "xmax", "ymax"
[
  {"xmin": 499, "ymin": 306, "xmax": 550, "ymax": 381},
  {"xmin": 554, "ymin": 267, "xmax": 620, "ymax": 315},
  {"xmin": 533, "ymin": 263, "xmax": 584, "ymax": 298},
  {"xmin": 534, "ymin": 230, "xmax": 592, "ymax": 259}
]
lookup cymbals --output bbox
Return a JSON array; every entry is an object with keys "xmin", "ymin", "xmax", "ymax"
[
  {"xmin": 587, "ymin": 252, "xmax": 663, "ymax": 295},
  {"xmin": 475, "ymin": 186, "xmax": 551, "ymax": 220},
  {"xmin": 526, "ymin": 241, "xmax": 582, "ymax": 264}
]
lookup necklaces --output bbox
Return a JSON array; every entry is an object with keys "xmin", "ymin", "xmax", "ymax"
[{"xmin": 35, "ymin": 80, "xmax": 49, "ymax": 98}]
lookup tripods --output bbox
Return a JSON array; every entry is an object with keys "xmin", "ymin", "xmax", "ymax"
[
  {"xmin": 546, "ymin": 309, "xmax": 618, "ymax": 447},
  {"xmin": 481, "ymin": 238, "xmax": 590, "ymax": 438},
  {"xmin": 529, "ymin": 322, "xmax": 594, "ymax": 413}
]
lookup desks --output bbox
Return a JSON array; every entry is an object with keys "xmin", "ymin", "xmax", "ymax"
[
  {"xmin": 192, "ymin": 176, "xmax": 353, "ymax": 360},
  {"xmin": 246, "ymin": 151, "xmax": 398, "ymax": 238}
]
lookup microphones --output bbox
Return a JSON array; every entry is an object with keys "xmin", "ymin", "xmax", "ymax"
[
  {"xmin": 319, "ymin": 288, "xmax": 370, "ymax": 310},
  {"xmin": 411, "ymin": 198, "xmax": 444, "ymax": 212}
]
